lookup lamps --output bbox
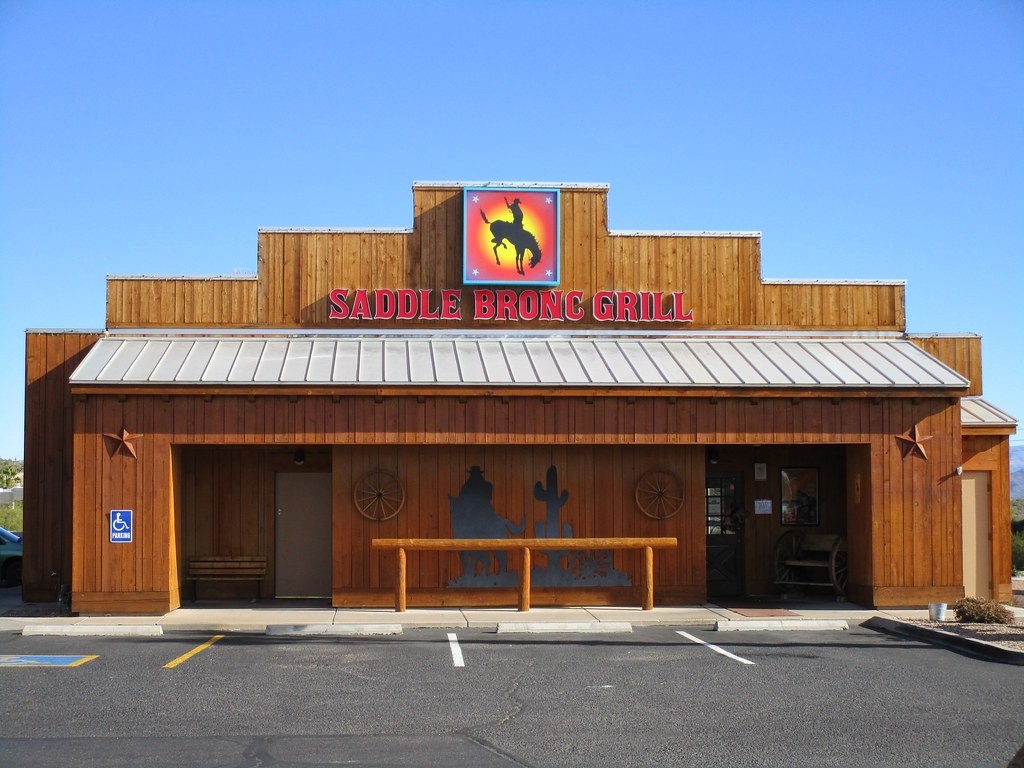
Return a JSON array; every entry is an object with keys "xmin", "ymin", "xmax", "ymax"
[
  {"xmin": 292, "ymin": 449, "xmax": 306, "ymax": 466},
  {"xmin": 709, "ymin": 448, "xmax": 720, "ymax": 465}
]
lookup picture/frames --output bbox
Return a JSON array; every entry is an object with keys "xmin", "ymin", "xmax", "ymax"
[
  {"xmin": 462, "ymin": 187, "xmax": 562, "ymax": 287},
  {"xmin": 780, "ymin": 466, "xmax": 820, "ymax": 527}
]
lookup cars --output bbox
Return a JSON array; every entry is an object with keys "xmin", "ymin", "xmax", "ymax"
[{"xmin": 0, "ymin": 526, "xmax": 23, "ymax": 586}]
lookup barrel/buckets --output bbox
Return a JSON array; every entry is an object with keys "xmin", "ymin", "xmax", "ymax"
[{"xmin": 929, "ymin": 603, "xmax": 947, "ymax": 621}]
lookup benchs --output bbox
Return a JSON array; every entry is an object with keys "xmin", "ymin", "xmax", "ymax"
[{"xmin": 186, "ymin": 555, "xmax": 267, "ymax": 604}]
